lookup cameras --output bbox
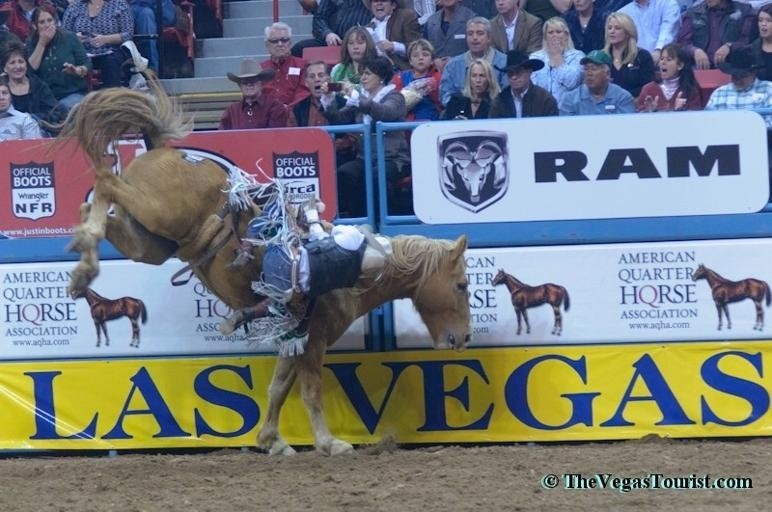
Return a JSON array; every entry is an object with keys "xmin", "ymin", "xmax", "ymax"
[{"xmin": 326, "ymin": 82, "xmax": 342, "ymax": 93}]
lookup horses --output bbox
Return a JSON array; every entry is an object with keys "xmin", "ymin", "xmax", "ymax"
[
  {"xmin": 492, "ymin": 268, "xmax": 569, "ymax": 335},
  {"xmin": 22, "ymin": 57, "xmax": 470, "ymax": 456},
  {"xmin": 692, "ymin": 264, "xmax": 772, "ymax": 331},
  {"xmin": 71, "ymin": 286, "xmax": 147, "ymax": 348}
]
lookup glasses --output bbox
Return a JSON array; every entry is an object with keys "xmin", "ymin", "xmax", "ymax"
[{"xmin": 268, "ymin": 37, "xmax": 290, "ymax": 43}]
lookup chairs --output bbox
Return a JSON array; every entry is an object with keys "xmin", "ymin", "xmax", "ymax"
[
  {"xmin": 302, "ymin": 46, "xmax": 342, "ymax": 67},
  {"xmin": 162, "ymin": 2, "xmax": 196, "ymax": 58},
  {"xmin": 694, "ymin": 69, "xmax": 734, "ymax": 108}
]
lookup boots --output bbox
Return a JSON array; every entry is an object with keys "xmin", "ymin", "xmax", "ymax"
[
  {"xmin": 220, "ymin": 307, "xmax": 254, "ymax": 335},
  {"xmin": 225, "ymin": 241, "xmax": 253, "ymax": 273}
]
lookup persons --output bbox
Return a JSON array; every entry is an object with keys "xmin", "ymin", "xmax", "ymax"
[
  {"xmin": 0, "ymin": 0, "xmax": 771, "ymax": 222},
  {"xmin": 218, "ymin": 175, "xmax": 393, "ymax": 358}
]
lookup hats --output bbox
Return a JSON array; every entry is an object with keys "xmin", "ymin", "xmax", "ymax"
[
  {"xmin": 719, "ymin": 43, "xmax": 765, "ymax": 77},
  {"xmin": 580, "ymin": 50, "xmax": 612, "ymax": 66},
  {"xmin": 226, "ymin": 57, "xmax": 276, "ymax": 83},
  {"xmin": 493, "ymin": 49, "xmax": 544, "ymax": 75}
]
